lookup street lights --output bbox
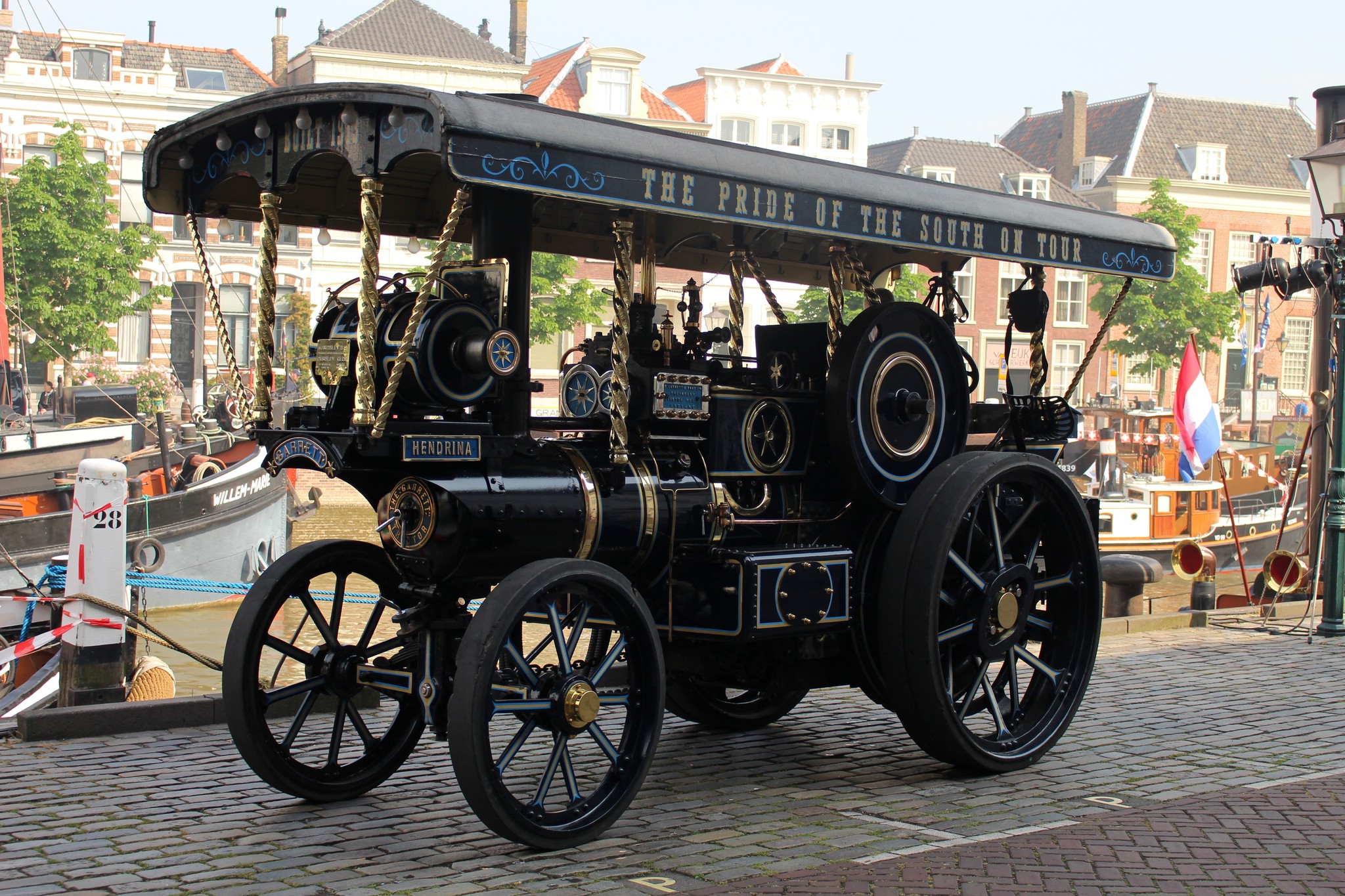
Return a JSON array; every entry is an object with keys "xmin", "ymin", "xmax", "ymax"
[
  {"xmin": 1298, "ymin": 117, "xmax": 1345, "ymax": 637},
  {"xmin": 1250, "ymin": 332, "xmax": 1288, "ymax": 445}
]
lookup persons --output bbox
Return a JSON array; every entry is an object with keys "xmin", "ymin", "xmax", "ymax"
[
  {"xmin": 37, "ymin": 381, "xmax": 58, "ymax": 415},
  {"xmin": 82, "ymin": 372, "xmax": 99, "ymax": 386},
  {"xmin": 1275, "ymin": 423, "xmax": 1302, "ymax": 441}
]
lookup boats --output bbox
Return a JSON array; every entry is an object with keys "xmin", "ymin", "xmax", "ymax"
[
  {"xmin": 0, "ymin": 412, "xmax": 288, "ymax": 639},
  {"xmin": 1055, "ymin": 385, "xmax": 1311, "ymax": 572}
]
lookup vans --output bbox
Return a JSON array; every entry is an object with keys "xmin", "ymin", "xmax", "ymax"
[{"xmin": 7, "ymin": 370, "xmax": 27, "ymax": 421}]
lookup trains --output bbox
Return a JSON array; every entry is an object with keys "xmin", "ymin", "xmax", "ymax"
[{"xmin": 143, "ymin": 83, "xmax": 1177, "ymax": 852}]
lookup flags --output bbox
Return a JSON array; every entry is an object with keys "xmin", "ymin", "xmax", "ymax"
[
  {"xmin": 1219, "ymin": 440, "xmax": 1235, "ymax": 455},
  {"xmin": 1236, "ymin": 294, "xmax": 1248, "ymax": 367},
  {"xmin": 1171, "ymin": 435, "xmax": 1180, "ymax": 443},
  {"xmin": 1145, "ymin": 434, "xmax": 1155, "ymax": 445},
  {"xmin": 1085, "ymin": 459, "xmax": 1125, "ymax": 482},
  {"xmin": 1158, "ymin": 434, "xmax": 1169, "ymax": 443},
  {"xmin": 1133, "ymin": 433, "xmax": 1141, "ymax": 444},
  {"xmin": 1075, "ymin": 430, "xmax": 1100, "ymax": 441},
  {"xmin": 1251, "ymin": 295, "xmax": 1271, "ymax": 356},
  {"xmin": 1279, "ymin": 487, "xmax": 1287, "ymax": 507},
  {"xmin": 1172, "ymin": 342, "xmax": 1223, "ymax": 482},
  {"xmin": 1119, "ymin": 432, "xmax": 1131, "ymax": 443},
  {"xmin": 1258, "ymin": 469, "xmax": 1286, "ymax": 491},
  {"xmin": 1238, "ymin": 454, "xmax": 1255, "ymax": 471}
]
